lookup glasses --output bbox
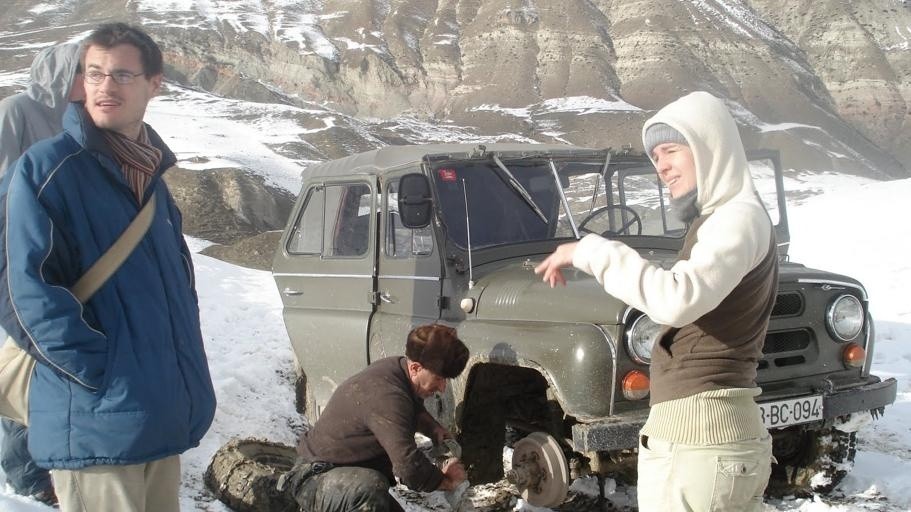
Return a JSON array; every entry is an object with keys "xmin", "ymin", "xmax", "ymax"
[{"xmin": 83, "ymin": 71, "xmax": 145, "ymax": 84}]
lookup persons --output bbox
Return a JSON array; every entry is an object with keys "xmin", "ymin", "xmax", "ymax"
[
  {"xmin": 7, "ymin": 24, "xmax": 216, "ymax": 512},
  {"xmin": 288, "ymin": 324, "xmax": 469, "ymax": 512},
  {"xmin": 534, "ymin": 91, "xmax": 777, "ymax": 512},
  {"xmin": 2, "ymin": 38, "xmax": 89, "ymax": 505}
]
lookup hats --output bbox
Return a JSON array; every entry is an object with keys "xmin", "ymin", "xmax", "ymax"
[
  {"xmin": 645, "ymin": 124, "xmax": 689, "ymax": 158},
  {"xmin": 406, "ymin": 324, "xmax": 469, "ymax": 378}
]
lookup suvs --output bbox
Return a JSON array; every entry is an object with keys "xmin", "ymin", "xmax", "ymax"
[{"xmin": 271, "ymin": 140, "xmax": 899, "ymax": 507}]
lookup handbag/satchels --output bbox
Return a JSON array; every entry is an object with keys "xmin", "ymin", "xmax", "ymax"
[{"xmin": 1, "ymin": 336, "xmax": 35, "ymax": 428}]
